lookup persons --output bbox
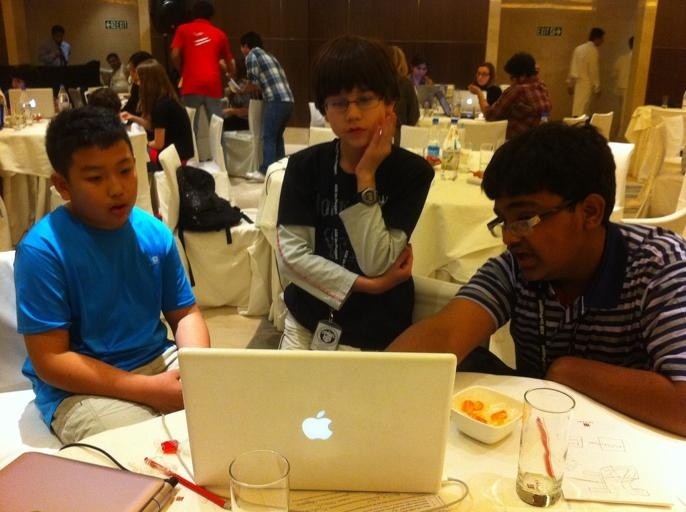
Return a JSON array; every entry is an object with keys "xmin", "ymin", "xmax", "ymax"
[
  {"xmin": 612, "ymin": 37, "xmax": 634, "ymax": 140},
  {"xmin": 276, "ymin": 35, "xmax": 435, "ymax": 352},
  {"xmin": 107, "ymin": 53, "xmax": 130, "ymax": 93},
  {"xmin": 170, "ymin": 1, "xmax": 235, "ymax": 135},
  {"xmin": 5, "ymin": 65, "xmax": 41, "ymax": 114},
  {"xmin": 235, "ymin": 31, "xmax": 295, "ymax": 183},
  {"xmin": 38, "ymin": 24, "xmax": 72, "ymax": 69},
  {"xmin": 120, "ymin": 50, "xmax": 194, "ymax": 172},
  {"xmin": 565, "ymin": 27, "xmax": 605, "ymax": 128},
  {"xmin": 13, "ymin": 104, "xmax": 212, "ymax": 445},
  {"xmin": 384, "ymin": 120, "xmax": 686, "ymax": 437},
  {"xmin": 384, "ymin": 44, "xmax": 552, "ymax": 141}
]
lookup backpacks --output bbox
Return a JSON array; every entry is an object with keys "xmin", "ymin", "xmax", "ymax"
[{"xmin": 176, "ymin": 165, "xmax": 241, "ymax": 232}]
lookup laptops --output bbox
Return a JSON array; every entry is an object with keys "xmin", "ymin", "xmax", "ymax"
[
  {"xmin": 178, "ymin": 346, "xmax": 457, "ymax": 495},
  {"xmin": 452, "ymin": 90, "xmax": 487, "ymax": 111},
  {"xmin": 68, "ymin": 88, "xmax": 88, "ymax": 108},
  {"xmin": 414, "ymin": 84, "xmax": 448, "ymax": 106},
  {"xmin": 0, "ymin": 452, "xmax": 178, "ymax": 512},
  {"xmin": 8, "ymin": 88, "xmax": 58, "ymax": 119},
  {"xmin": 435, "ymin": 90, "xmax": 474, "ymax": 119}
]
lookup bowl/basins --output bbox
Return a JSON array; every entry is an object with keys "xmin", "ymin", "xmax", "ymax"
[{"xmin": 451, "ymin": 385, "xmax": 526, "ymax": 444}]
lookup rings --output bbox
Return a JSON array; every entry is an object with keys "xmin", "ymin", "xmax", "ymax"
[
  {"xmin": 377, "ymin": 127, "xmax": 384, "ymax": 136},
  {"xmin": 390, "ymin": 134, "xmax": 397, "ymax": 145}
]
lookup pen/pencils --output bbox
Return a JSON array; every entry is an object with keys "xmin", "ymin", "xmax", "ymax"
[{"xmin": 141, "ymin": 456, "xmax": 232, "ymax": 511}]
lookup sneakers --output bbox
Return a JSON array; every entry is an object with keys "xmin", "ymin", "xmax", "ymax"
[{"xmin": 246, "ymin": 171, "xmax": 265, "ymax": 183}]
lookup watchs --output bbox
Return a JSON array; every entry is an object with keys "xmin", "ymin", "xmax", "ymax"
[{"xmin": 357, "ymin": 188, "xmax": 380, "ymax": 206}]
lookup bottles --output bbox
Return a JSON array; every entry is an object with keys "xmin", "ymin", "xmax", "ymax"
[
  {"xmin": 57, "ymin": 85, "xmax": 69, "ymax": 113},
  {"xmin": 4, "ymin": 80, "xmax": 39, "ymax": 132},
  {"xmin": 424, "ymin": 119, "xmax": 443, "ymax": 167},
  {"xmin": 458, "ymin": 124, "xmax": 471, "ymax": 172},
  {"xmin": 442, "ymin": 115, "xmax": 465, "ymax": 183}
]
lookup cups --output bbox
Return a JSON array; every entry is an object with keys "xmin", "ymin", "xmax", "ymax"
[
  {"xmin": 226, "ymin": 450, "xmax": 291, "ymax": 511},
  {"xmin": 479, "ymin": 142, "xmax": 494, "ymax": 177},
  {"xmin": 521, "ymin": 388, "xmax": 576, "ymax": 508}
]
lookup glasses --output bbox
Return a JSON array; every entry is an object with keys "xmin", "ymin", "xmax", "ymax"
[
  {"xmin": 319, "ymin": 94, "xmax": 385, "ymax": 112},
  {"xmin": 488, "ymin": 198, "xmax": 574, "ymax": 238}
]
